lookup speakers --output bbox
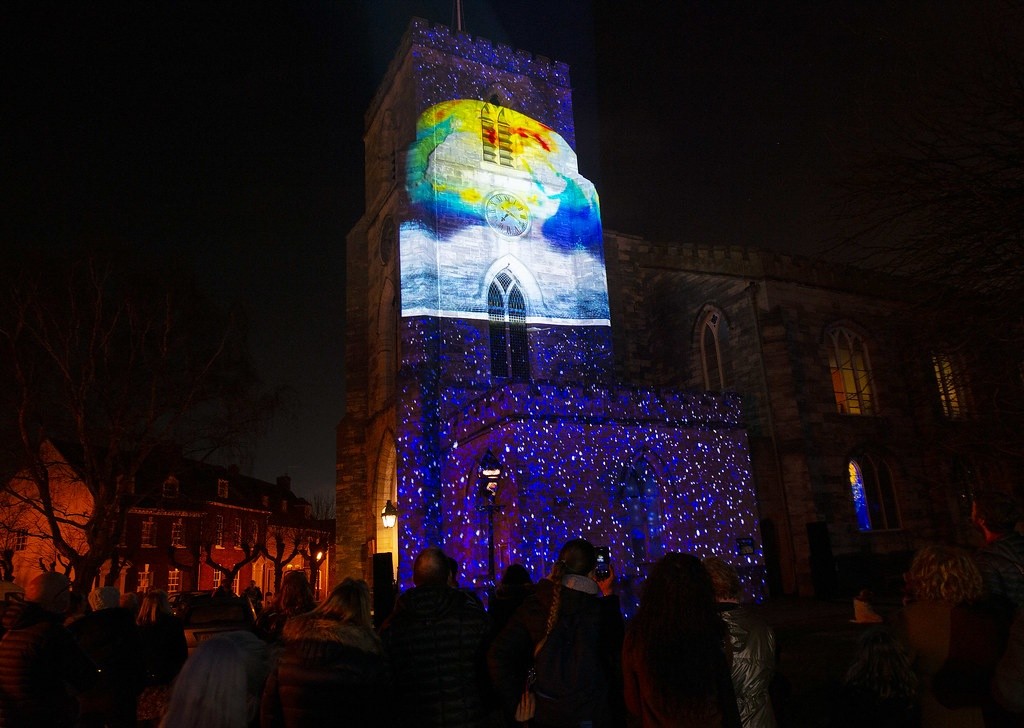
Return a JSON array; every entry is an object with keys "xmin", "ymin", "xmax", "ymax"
[
  {"xmin": 373, "ymin": 552, "xmax": 394, "ymax": 628},
  {"xmin": 804, "ymin": 521, "xmax": 842, "ymax": 600}
]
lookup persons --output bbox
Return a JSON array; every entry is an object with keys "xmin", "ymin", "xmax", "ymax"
[{"xmin": 0, "ymin": 490, "xmax": 1024, "ymax": 728}]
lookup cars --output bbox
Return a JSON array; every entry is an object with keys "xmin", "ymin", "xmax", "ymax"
[{"xmin": 169, "ymin": 589, "xmax": 257, "ymax": 659}]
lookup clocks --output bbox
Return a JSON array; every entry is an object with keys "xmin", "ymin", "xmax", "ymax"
[{"xmin": 484, "ymin": 190, "xmax": 533, "ymax": 239}]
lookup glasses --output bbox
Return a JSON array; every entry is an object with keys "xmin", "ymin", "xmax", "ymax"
[{"xmin": 53, "ymin": 580, "xmax": 74, "ymax": 600}]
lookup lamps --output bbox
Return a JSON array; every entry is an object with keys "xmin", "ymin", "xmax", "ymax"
[{"xmin": 380, "ymin": 499, "xmax": 398, "ymax": 528}]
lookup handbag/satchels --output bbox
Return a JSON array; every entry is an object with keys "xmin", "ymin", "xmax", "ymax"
[{"xmin": 520, "ymin": 665, "xmax": 536, "ymax": 695}]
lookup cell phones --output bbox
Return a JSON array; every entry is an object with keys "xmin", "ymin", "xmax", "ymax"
[{"xmin": 594, "ymin": 545, "xmax": 609, "ymax": 580}]
